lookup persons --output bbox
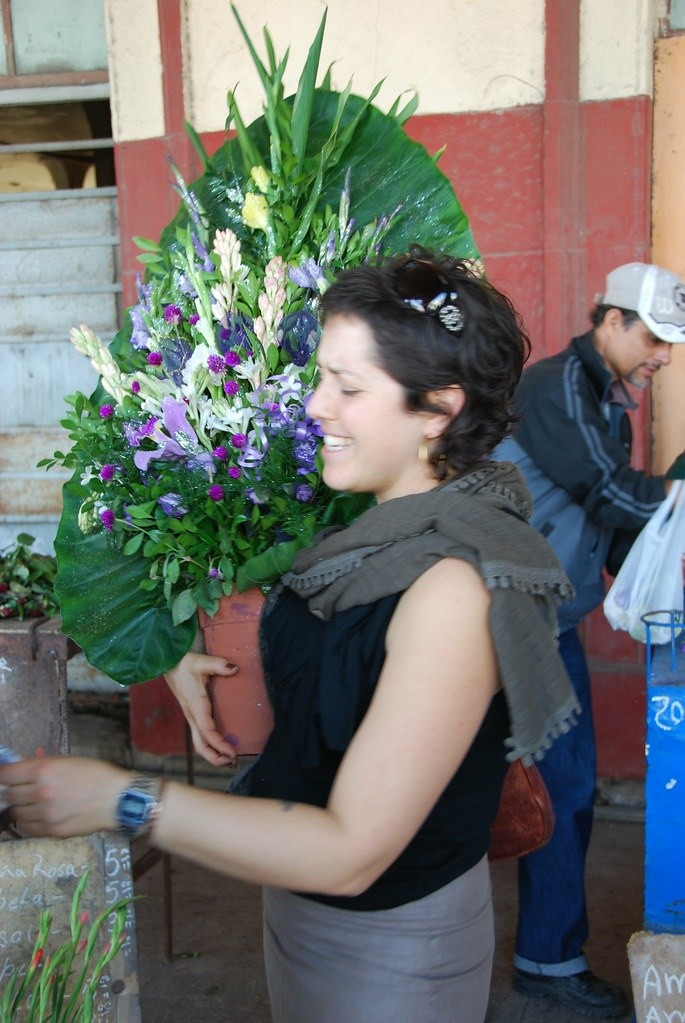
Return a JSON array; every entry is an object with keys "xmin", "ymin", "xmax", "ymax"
[
  {"xmin": 0, "ymin": 240, "xmax": 576, "ymax": 1023},
  {"xmin": 495, "ymin": 262, "xmax": 685, "ymax": 1017}
]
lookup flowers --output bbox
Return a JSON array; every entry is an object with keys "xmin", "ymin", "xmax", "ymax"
[{"xmin": 65, "ymin": 0, "xmax": 463, "ymax": 594}]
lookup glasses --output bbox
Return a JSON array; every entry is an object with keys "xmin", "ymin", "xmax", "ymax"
[{"xmin": 394, "ymin": 259, "xmax": 467, "ymax": 342}]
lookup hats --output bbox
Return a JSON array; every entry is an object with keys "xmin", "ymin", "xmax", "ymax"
[{"xmin": 594, "ymin": 262, "xmax": 685, "ymax": 343}]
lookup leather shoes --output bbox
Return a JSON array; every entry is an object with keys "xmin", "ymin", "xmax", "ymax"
[{"xmin": 513, "ymin": 966, "xmax": 628, "ymax": 1017}]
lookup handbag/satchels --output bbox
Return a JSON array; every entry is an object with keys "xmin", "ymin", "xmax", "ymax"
[
  {"xmin": 602, "ymin": 479, "xmax": 685, "ymax": 644},
  {"xmin": 488, "ymin": 753, "xmax": 555, "ymax": 863}
]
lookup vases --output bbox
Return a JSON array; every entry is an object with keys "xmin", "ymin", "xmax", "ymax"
[{"xmin": 196, "ymin": 584, "xmax": 278, "ymax": 760}]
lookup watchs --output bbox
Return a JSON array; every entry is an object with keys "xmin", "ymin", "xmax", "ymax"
[{"xmin": 114, "ymin": 771, "xmax": 164, "ymax": 839}]
